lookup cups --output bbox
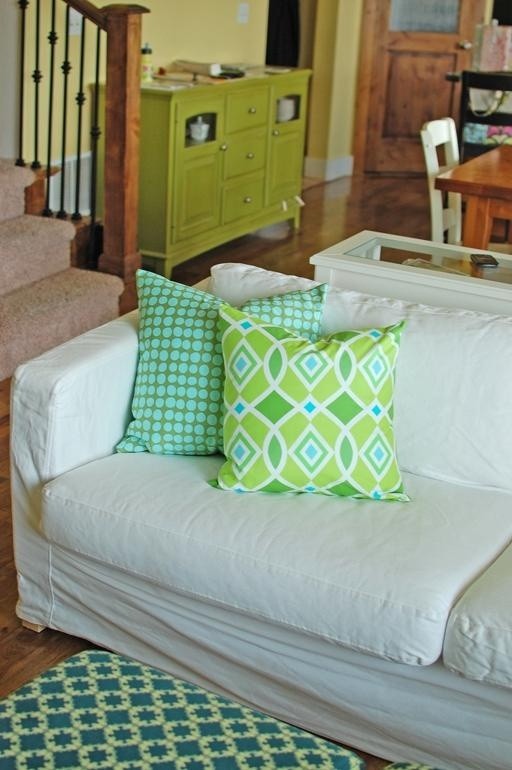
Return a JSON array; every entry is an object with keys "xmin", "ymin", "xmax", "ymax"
[{"xmin": 191, "ymin": 123, "xmax": 210, "ymax": 143}]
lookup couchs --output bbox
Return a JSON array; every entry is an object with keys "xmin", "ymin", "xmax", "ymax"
[{"xmin": 2, "ymin": 259, "xmax": 511, "ymax": 769}]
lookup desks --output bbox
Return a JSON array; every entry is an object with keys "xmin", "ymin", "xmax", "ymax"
[{"xmin": 437, "ymin": 143, "xmax": 512, "ymax": 253}]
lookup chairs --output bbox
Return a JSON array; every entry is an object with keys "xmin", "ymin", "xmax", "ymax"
[{"xmin": 418, "ymin": 115, "xmax": 512, "ymax": 255}]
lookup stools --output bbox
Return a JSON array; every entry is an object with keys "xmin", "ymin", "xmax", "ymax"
[{"xmin": 1, "ymin": 646, "xmax": 362, "ymax": 770}]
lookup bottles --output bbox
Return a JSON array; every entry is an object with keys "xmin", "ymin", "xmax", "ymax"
[{"xmin": 141, "ymin": 47, "xmax": 152, "ymax": 84}]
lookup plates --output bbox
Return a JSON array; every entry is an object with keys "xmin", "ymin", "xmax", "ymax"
[{"xmin": 275, "ymin": 99, "xmax": 296, "ymax": 123}]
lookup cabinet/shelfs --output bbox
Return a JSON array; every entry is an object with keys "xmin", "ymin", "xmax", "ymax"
[
  {"xmin": 220, "ymin": 77, "xmax": 272, "ymax": 244},
  {"xmin": 303, "ymin": 229, "xmax": 512, "ymax": 315},
  {"xmin": 265, "ymin": 67, "xmax": 310, "ymax": 228},
  {"xmin": 88, "ymin": 64, "xmax": 228, "ymax": 283},
  {"xmin": 447, "ymin": 70, "xmax": 512, "ymax": 211}
]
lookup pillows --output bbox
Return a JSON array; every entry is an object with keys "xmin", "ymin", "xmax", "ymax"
[
  {"xmin": 208, "ymin": 299, "xmax": 413, "ymax": 508},
  {"xmin": 114, "ymin": 271, "xmax": 334, "ymax": 461}
]
